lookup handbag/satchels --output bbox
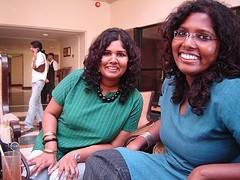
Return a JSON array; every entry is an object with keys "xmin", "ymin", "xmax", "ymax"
[
  {"xmin": 0, "ymin": 138, "xmax": 30, "ymax": 180},
  {"xmin": 34, "ymin": 54, "xmax": 45, "ymax": 73}
]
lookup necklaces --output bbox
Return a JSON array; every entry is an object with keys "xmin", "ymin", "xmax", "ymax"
[{"xmin": 95, "ymin": 75, "xmax": 122, "ymax": 103}]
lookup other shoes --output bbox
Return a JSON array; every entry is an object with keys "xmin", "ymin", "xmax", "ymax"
[
  {"xmin": 21, "ymin": 128, "xmax": 33, "ymax": 133},
  {"xmin": 33, "ymin": 123, "xmax": 40, "ymax": 127}
]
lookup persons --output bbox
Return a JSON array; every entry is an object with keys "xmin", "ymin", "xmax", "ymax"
[
  {"xmin": 40, "ymin": 52, "xmax": 59, "ymax": 104},
  {"xmin": 21, "ymin": 28, "xmax": 143, "ymax": 180},
  {"xmin": 21, "ymin": 40, "xmax": 49, "ymax": 132},
  {"xmin": 83, "ymin": 0, "xmax": 240, "ymax": 180}
]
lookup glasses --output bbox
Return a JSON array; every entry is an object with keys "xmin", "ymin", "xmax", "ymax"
[{"xmin": 174, "ymin": 30, "xmax": 219, "ymax": 42}]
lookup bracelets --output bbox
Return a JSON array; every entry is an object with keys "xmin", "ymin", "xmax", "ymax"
[
  {"xmin": 42, "ymin": 132, "xmax": 57, "ymax": 140},
  {"xmin": 44, "ymin": 149, "xmax": 58, "ymax": 153},
  {"xmin": 42, "ymin": 138, "xmax": 58, "ymax": 145},
  {"xmin": 138, "ymin": 131, "xmax": 154, "ymax": 150}
]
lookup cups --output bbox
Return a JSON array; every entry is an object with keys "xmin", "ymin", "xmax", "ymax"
[{"xmin": 1, "ymin": 151, "xmax": 21, "ymax": 180}]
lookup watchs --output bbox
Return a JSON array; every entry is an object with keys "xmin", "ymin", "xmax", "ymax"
[{"xmin": 71, "ymin": 150, "xmax": 81, "ymax": 163}]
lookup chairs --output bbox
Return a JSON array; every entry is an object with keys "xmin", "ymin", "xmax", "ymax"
[
  {"xmin": 0, "ymin": 51, "xmax": 22, "ymax": 151},
  {"xmin": 37, "ymin": 91, "xmax": 155, "ymax": 145}
]
